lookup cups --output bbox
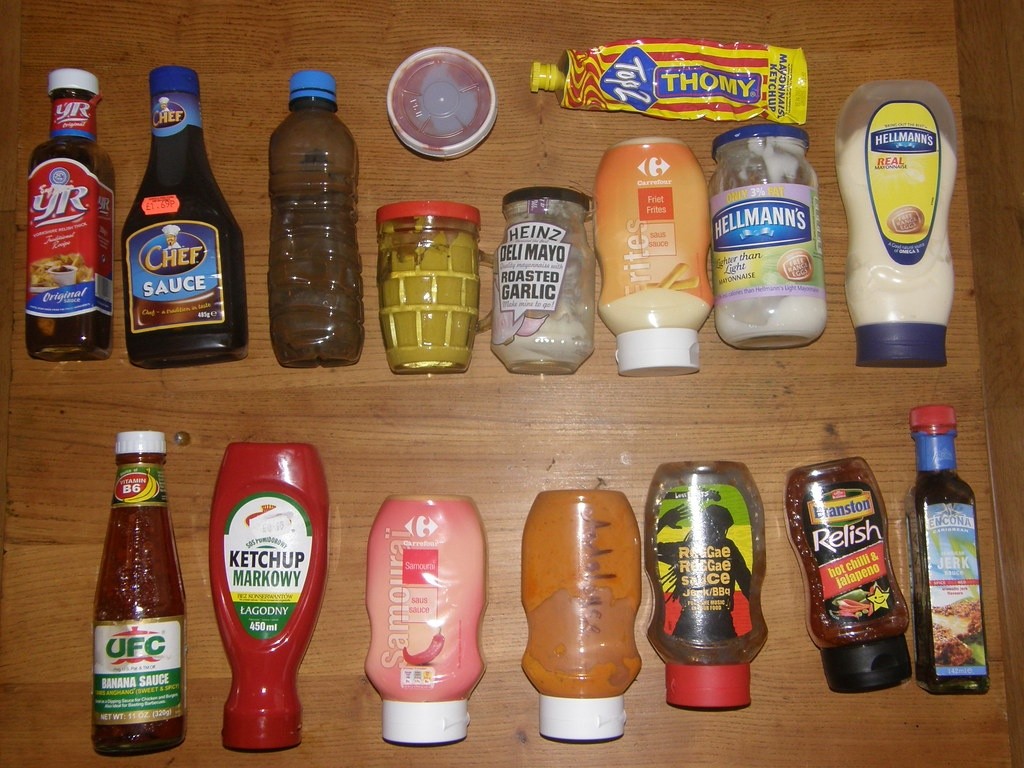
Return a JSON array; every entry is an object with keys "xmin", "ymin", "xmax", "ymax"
[
  {"xmin": 387, "ymin": 47, "xmax": 497, "ymax": 160},
  {"xmin": 48, "ymin": 266, "xmax": 77, "ymax": 286},
  {"xmin": 372, "ymin": 202, "xmax": 495, "ymax": 376}
]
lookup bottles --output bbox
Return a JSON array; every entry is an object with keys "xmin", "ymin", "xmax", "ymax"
[
  {"xmin": 644, "ymin": 461, "xmax": 768, "ymax": 708},
  {"xmin": 785, "ymin": 458, "xmax": 912, "ymax": 693},
  {"xmin": 835, "ymin": 80, "xmax": 956, "ymax": 366},
  {"xmin": 267, "ymin": 70, "xmax": 365, "ymax": 368},
  {"xmin": 905, "ymin": 405, "xmax": 989, "ymax": 695},
  {"xmin": 365, "ymin": 494, "xmax": 487, "ymax": 743},
  {"xmin": 522, "ymin": 489, "xmax": 641, "ymax": 740},
  {"xmin": 26, "ymin": 69, "xmax": 114, "ymax": 363},
  {"xmin": 91, "ymin": 431, "xmax": 186, "ymax": 754},
  {"xmin": 594, "ymin": 137, "xmax": 713, "ymax": 378},
  {"xmin": 209, "ymin": 443, "xmax": 329, "ymax": 749},
  {"xmin": 122, "ymin": 66, "xmax": 248, "ymax": 369}
]
